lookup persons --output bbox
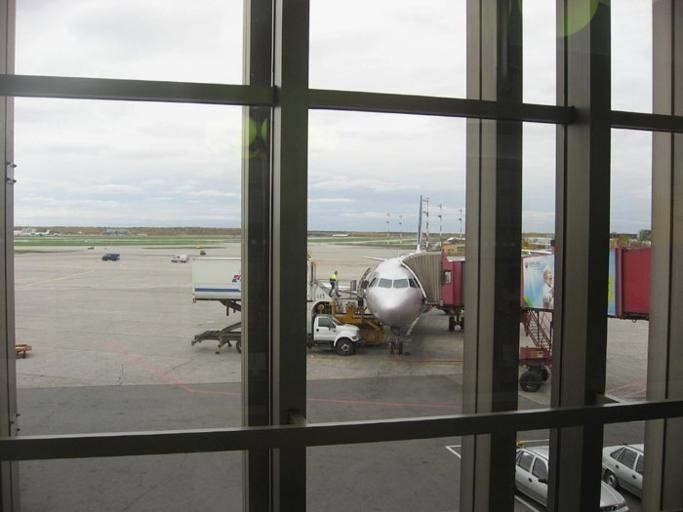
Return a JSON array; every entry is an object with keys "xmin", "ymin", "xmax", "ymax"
[
  {"xmin": 541, "ymin": 266, "xmax": 554, "ymax": 311},
  {"xmin": 328, "ymin": 270, "xmax": 342, "ymax": 297}
]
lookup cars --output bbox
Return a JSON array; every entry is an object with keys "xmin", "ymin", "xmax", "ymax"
[
  {"xmin": 602, "ymin": 442, "xmax": 643, "ymax": 500},
  {"xmin": 516, "ymin": 445, "xmax": 630, "ymax": 512}
]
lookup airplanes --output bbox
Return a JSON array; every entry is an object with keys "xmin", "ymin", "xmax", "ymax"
[{"xmin": 354, "ymin": 193, "xmax": 442, "ymax": 356}]
